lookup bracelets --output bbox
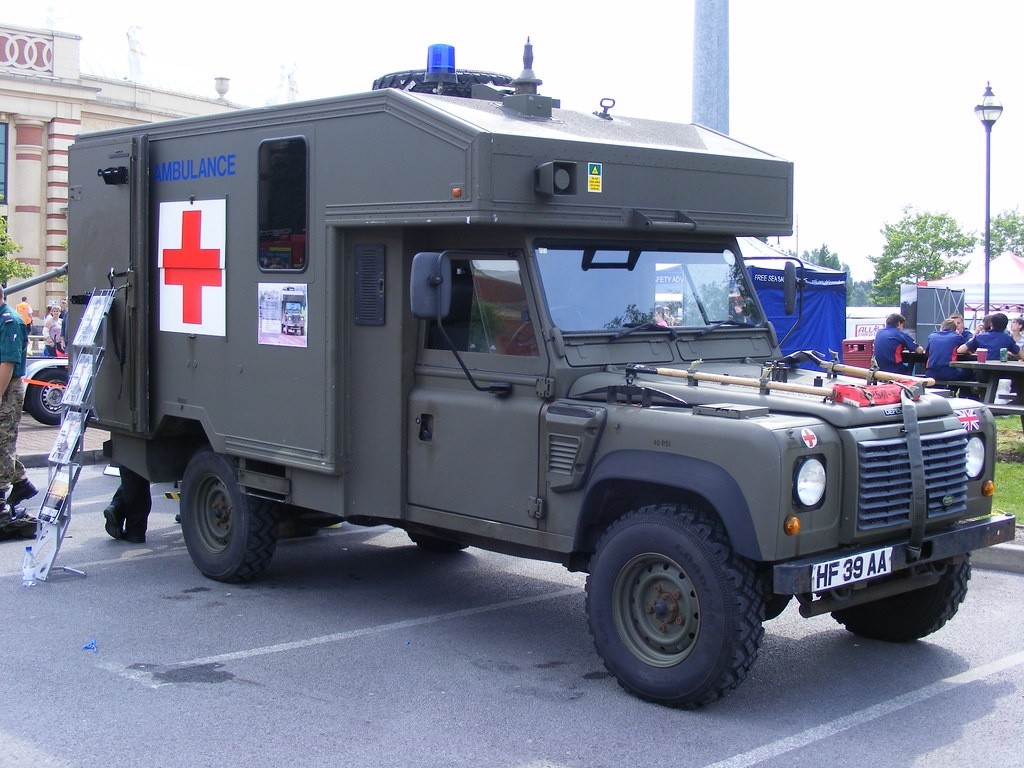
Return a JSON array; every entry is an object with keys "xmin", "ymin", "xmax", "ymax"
[{"xmin": 31, "ymin": 320, "xmax": 33, "ymax": 323}]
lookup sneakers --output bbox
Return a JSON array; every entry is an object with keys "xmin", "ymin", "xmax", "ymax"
[{"xmin": 7, "ymin": 479, "xmax": 39, "ymax": 506}]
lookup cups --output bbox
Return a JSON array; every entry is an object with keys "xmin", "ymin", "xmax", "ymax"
[
  {"xmin": 1000, "ymin": 348, "xmax": 1008, "ymax": 361},
  {"xmin": 976, "ymin": 348, "xmax": 988, "ymax": 363}
]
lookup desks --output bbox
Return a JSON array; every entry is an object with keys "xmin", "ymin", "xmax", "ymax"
[
  {"xmin": 901, "ymin": 350, "xmax": 977, "ymax": 364},
  {"xmin": 950, "ymin": 359, "xmax": 1024, "ymax": 404}
]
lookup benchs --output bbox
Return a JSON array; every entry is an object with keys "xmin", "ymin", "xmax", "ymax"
[
  {"xmin": 985, "ymin": 403, "xmax": 1024, "ymax": 416},
  {"xmin": 933, "ymin": 379, "xmax": 988, "ymax": 388},
  {"xmin": 998, "ymin": 393, "xmax": 1017, "ymax": 401}
]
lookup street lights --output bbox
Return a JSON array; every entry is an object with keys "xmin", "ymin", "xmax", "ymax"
[{"xmin": 973, "ymin": 81, "xmax": 1004, "ymax": 316}]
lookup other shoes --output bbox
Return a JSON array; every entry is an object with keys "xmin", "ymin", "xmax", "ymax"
[
  {"xmin": 104, "ymin": 505, "xmax": 124, "ymax": 540},
  {"xmin": 123, "ymin": 529, "xmax": 146, "ymax": 543}
]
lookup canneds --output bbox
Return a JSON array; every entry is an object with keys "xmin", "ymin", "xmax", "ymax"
[{"xmin": 1000, "ymin": 348, "xmax": 1007, "ymax": 362}]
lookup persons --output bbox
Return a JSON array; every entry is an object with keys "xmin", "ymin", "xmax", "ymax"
[
  {"xmin": 43, "ymin": 305, "xmax": 53, "ymax": 324},
  {"xmin": 954, "ymin": 314, "xmax": 1024, "ymax": 363},
  {"xmin": 15, "ymin": 297, "xmax": 34, "ymax": 334},
  {"xmin": 944, "ymin": 315, "xmax": 973, "ymax": 398},
  {"xmin": 60, "ymin": 297, "xmax": 69, "ymax": 355},
  {"xmin": 58, "ymin": 299, "xmax": 66, "ymax": 319},
  {"xmin": 921, "ymin": 318, "xmax": 972, "ymax": 400},
  {"xmin": 970, "ymin": 315, "xmax": 993, "ymax": 339},
  {"xmin": 104, "ymin": 465, "xmax": 153, "ymax": 544},
  {"xmin": 1006, "ymin": 319, "xmax": 1024, "ymax": 361},
  {"xmin": 873, "ymin": 313, "xmax": 924, "ymax": 375},
  {"xmin": 655, "ymin": 305, "xmax": 674, "ymax": 327},
  {"xmin": 42, "ymin": 307, "xmax": 63, "ymax": 356},
  {"xmin": 0, "ymin": 285, "xmax": 39, "ymax": 507}
]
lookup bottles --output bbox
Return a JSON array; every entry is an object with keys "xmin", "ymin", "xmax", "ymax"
[{"xmin": 22, "ymin": 546, "xmax": 36, "ymax": 586}]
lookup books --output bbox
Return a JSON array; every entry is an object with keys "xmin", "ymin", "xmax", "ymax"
[
  {"xmin": 47, "ymin": 410, "xmax": 84, "ymax": 463},
  {"xmin": 60, "ymin": 353, "xmax": 97, "ymax": 406},
  {"xmin": 71, "ymin": 296, "xmax": 114, "ymax": 346},
  {"xmin": 38, "ymin": 463, "xmax": 77, "ymax": 524}
]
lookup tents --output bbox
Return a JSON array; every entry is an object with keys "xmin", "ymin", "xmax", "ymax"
[
  {"xmin": 886, "ymin": 250, "xmax": 1024, "ymax": 332},
  {"xmin": 656, "ymin": 237, "xmax": 847, "ymax": 377}
]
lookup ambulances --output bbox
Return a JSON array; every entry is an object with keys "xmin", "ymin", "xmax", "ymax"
[{"xmin": 67, "ymin": 37, "xmax": 1017, "ymax": 713}]
